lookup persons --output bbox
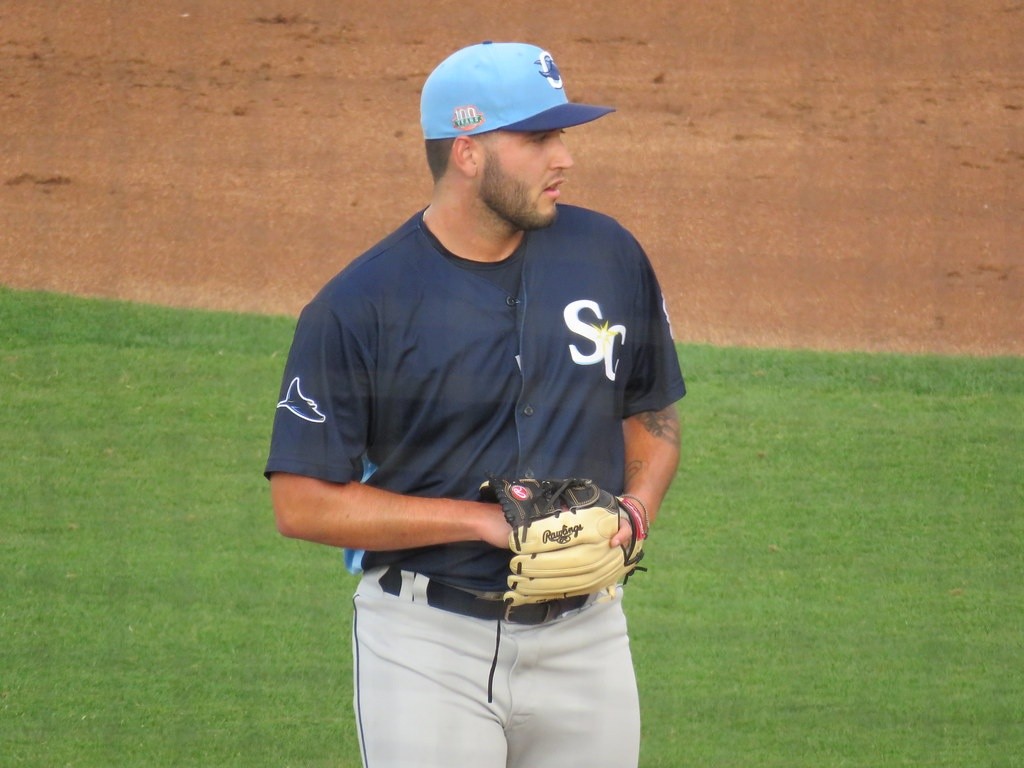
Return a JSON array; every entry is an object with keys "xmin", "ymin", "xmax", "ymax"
[{"xmin": 263, "ymin": 41, "xmax": 685, "ymax": 768}]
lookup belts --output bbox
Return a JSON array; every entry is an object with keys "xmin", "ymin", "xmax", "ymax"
[{"xmin": 379, "ymin": 568, "xmax": 589, "ymax": 625}]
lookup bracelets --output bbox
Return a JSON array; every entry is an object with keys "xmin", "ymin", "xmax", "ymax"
[{"xmin": 621, "ymin": 494, "xmax": 650, "ymax": 531}]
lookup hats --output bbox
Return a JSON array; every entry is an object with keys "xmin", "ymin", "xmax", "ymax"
[{"xmin": 420, "ymin": 41, "xmax": 617, "ymax": 139}]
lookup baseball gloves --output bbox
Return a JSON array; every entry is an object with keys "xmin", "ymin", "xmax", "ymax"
[{"xmin": 478, "ymin": 475, "xmax": 647, "ymax": 606}]
